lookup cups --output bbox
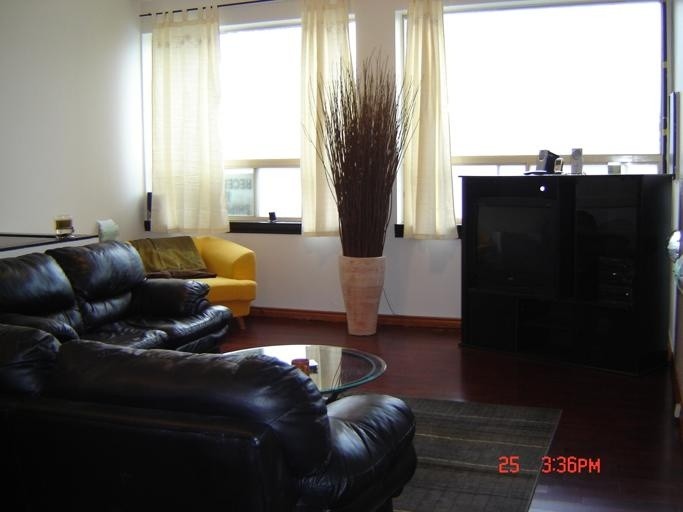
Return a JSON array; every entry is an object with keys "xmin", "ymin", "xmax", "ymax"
[
  {"xmin": 56, "ymin": 214, "xmax": 72, "ymax": 240},
  {"xmin": 608, "ymin": 161, "xmax": 621, "ymax": 175}
]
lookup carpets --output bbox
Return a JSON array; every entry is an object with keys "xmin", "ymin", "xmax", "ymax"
[{"xmin": 331, "ymin": 391, "xmax": 563, "ymax": 510}]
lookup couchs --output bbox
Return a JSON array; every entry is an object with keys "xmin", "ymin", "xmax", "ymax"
[{"xmin": 0, "ymin": 239, "xmax": 418, "ymax": 512}]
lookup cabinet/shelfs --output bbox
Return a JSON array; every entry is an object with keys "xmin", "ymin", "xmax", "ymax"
[{"xmin": 458, "ymin": 173, "xmax": 674, "ymax": 376}]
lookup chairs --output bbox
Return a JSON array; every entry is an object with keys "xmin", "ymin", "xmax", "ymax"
[{"xmin": 128, "ymin": 235, "xmax": 257, "ymax": 331}]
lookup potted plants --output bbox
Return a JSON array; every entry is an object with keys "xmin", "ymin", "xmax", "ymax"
[{"xmin": 316, "ymin": 45, "xmax": 410, "ymax": 336}]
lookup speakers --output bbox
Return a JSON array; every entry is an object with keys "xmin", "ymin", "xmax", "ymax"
[
  {"xmin": 572, "ymin": 148, "xmax": 583, "ymax": 174},
  {"xmin": 536, "ymin": 150, "xmax": 559, "ymax": 172}
]
